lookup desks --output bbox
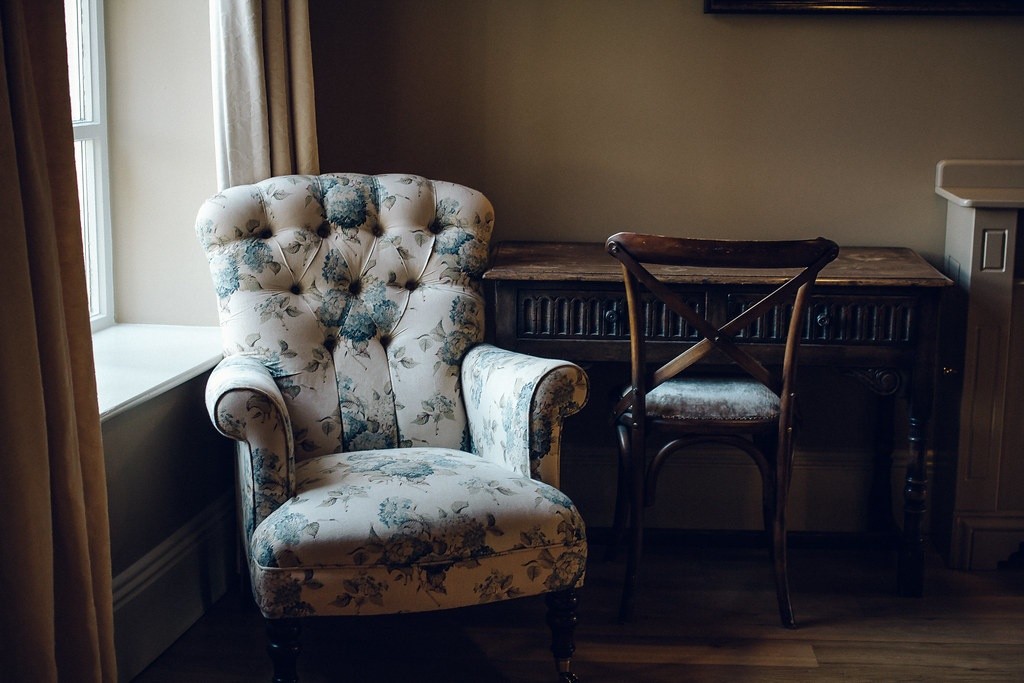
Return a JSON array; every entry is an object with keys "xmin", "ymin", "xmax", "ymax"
[{"xmin": 483, "ymin": 240, "xmax": 956, "ymax": 598}]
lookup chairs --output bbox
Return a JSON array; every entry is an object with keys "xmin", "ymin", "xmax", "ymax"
[
  {"xmin": 193, "ymin": 171, "xmax": 590, "ymax": 683},
  {"xmin": 602, "ymin": 232, "xmax": 840, "ymax": 630}
]
